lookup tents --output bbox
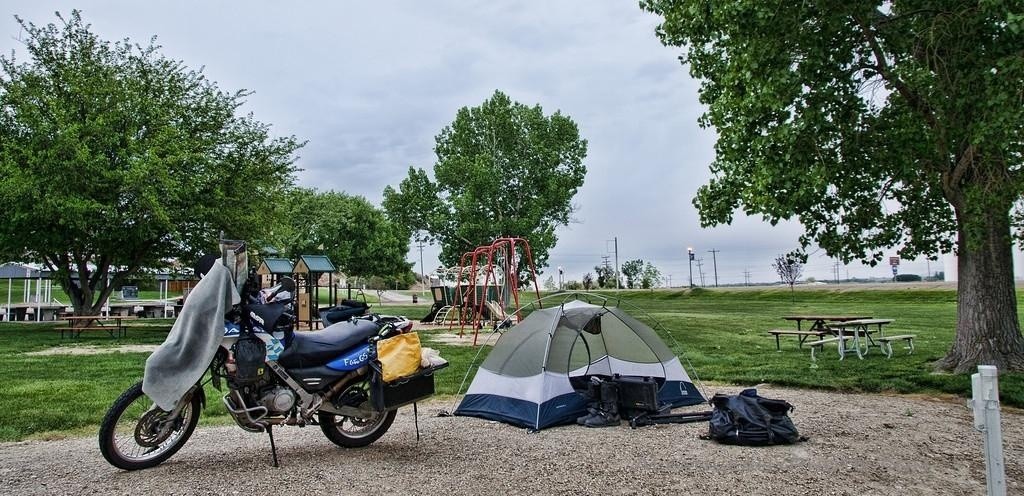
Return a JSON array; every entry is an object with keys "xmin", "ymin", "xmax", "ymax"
[{"xmin": 446, "ymin": 290, "xmax": 713, "ymax": 433}]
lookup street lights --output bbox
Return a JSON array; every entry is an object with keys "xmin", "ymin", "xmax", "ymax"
[{"xmin": 688, "ymin": 247, "xmax": 692, "ymax": 288}]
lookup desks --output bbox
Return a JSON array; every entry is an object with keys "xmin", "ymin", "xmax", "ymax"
[
  {"xmin": 780, "ymin": 308, "xmax": 895, "ymax": 364},
  {"xmin": 54, "ymin": 314, "xmax": 141, "ymax": 343}
]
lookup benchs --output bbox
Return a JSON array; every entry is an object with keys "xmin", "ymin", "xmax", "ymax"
[
  {"xmin": 873, "ymin": 333, "xmax": 919, "ymax": 361},
  {"xmin": 801, "ymin": 334, "xmax": 853, "ymax": 367},
  {"xmin": 56, "ymin": 324, "xmax": 132, "ymax": 343},
  {"xmin": 768, "ymin": 327, "xmax": 877, "ymax": 354}
]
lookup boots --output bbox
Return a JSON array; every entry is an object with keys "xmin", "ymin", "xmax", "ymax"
[{"xmin": 577, "ymin": 380, "xmax": 621, "ymax": 427}]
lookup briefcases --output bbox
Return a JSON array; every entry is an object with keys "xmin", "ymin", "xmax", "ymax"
[{"xmin": 368, "ymin": 370, "xmax": 434, "ymax": 412}]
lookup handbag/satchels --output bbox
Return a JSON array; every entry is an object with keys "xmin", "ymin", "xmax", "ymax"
[
  {"xmin": 700, "ymin": 389, "xmax": 810, "ymax": 447},
  {"xmin": 377, "ymin": 333, "xmax": 423, "ymax": 383},
  {"xmin": 234, "ymin": 307, "xmax": 267, "ymax": 383}
]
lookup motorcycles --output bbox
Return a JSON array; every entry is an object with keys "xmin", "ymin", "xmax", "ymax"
[{"xmin": 99, "ymin": 228, "xmax": 450, "ymax": 470}]
